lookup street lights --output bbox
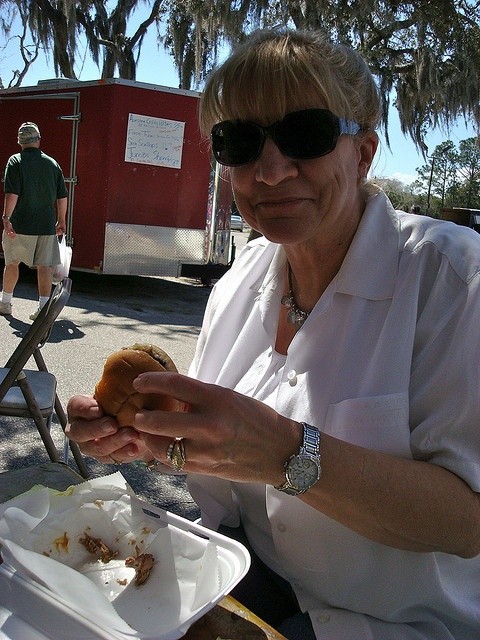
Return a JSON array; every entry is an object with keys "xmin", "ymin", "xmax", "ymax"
[{"xmin": 422, "ymin": 141, "xmax": 451, "ymax": 215}]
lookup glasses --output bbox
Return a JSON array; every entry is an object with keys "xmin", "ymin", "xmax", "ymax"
[{"xmin": 210, "ymin": 108, "xmax": 365, "ymax": 167}]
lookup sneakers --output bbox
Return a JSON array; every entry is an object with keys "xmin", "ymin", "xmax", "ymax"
[
  {"xmin": 29, "ymin": 308, "xmax": 42, "ymax": 319},
  {"xmin": 0, "ymin": 300, "xmax": 12, "ymax": 315}
]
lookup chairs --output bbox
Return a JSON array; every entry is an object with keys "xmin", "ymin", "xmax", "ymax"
[{"xmin": 1, "ymin": 276, "xmax": 90, "ymax": 478}]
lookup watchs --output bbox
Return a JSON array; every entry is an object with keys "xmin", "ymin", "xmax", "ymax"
[{"xmin": 274, "ymin": 422, "xmax": 323, "ymax": 497}]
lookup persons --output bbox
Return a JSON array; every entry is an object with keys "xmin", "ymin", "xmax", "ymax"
[
  {"xmin": 0, "ymin": 122, "xmax": 69, "ymax": 322},
  {"xmin": 64, "ymin": 27, "xmax": 479, "ymax": 640}
]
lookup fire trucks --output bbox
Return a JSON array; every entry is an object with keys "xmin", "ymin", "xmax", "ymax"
[{"xmin": 0, "ymin": 78, "xmax": 235, "ymax": 285}]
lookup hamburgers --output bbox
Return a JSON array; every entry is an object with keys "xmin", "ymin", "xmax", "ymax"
[{"xmin": 91, "ymin": 343, "xmax": 185, "ymax": 437}]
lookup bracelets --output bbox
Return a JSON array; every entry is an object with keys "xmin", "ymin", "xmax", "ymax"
[{"xmin": 1, "ymin": 216, "xmax": 11, "ymax": 220}]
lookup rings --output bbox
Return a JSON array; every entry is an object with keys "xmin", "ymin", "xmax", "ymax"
[{"xmin": 164, "ymin": 438, "xmax": 185, "ymax": 472}]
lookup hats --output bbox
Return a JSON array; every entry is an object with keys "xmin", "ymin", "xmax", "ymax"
[{"xmin": 18, "ymin": 122, "xmax": 41, "ymax": 144}]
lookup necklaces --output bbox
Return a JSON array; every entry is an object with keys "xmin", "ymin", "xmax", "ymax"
[{"xmin": 281, "ymin": 259, "xmax": 310, "ymax": 327}]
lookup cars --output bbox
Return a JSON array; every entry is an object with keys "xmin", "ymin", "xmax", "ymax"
[{"xmin": 231, "ymin": 215, "xmax": 244, "ymax": 232}]
